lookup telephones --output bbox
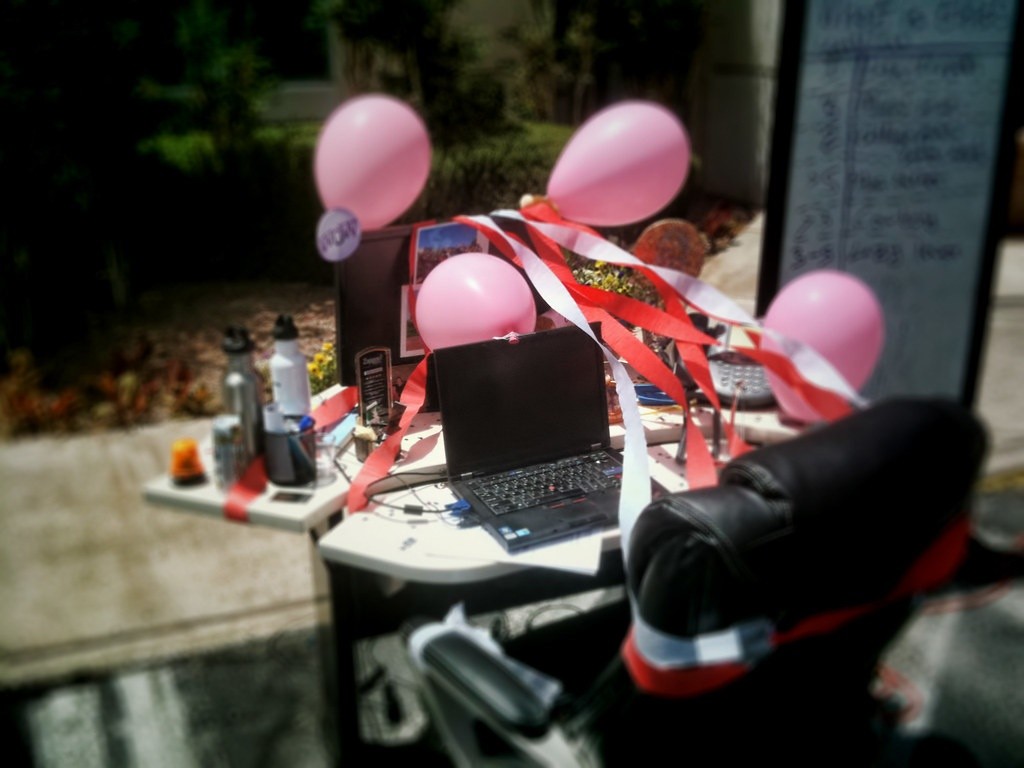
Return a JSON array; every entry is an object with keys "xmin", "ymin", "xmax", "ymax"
[{"xmin": 674, "ymin": 300, "xmax": 776, "ymax": 410}]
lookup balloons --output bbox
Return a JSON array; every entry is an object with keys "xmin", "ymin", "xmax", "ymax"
[
  {"xmin": 314, "ymin": 95, "xmax": 432, "ymax": 231},
  {"xmin": 416, "ymin": 252, "xmax": 536, "ymax": 352},
  {"xmin": 545, "ymin": 102, "xmax": 688, "ymax": 226},
  {"xmin": 760, "ymin": 269, "xmax": 882, "ymax": 421}
]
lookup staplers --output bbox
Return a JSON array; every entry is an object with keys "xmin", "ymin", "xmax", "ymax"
[{"xmin": 634, "ymin": 384, "xmax": 678, "ymax": 406}]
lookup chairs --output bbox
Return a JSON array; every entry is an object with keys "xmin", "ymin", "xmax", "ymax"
[{"xmin": 398, "ymin": 396, "xmax": 991, "ymax": 768}]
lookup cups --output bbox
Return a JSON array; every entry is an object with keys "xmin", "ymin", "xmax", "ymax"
[
  {"xmin": 257, "ymin": 415, "xmax": 316, "ymax": 486},
  {"xmin": 352, "ymin": 426, "xmax": 402, "ymax": 464},
  {"xmin": 315, "ymin": 434, "xmax": 338, "ymax": 485}
]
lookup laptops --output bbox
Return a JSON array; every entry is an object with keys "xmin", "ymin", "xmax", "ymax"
[{"xmin": 432, "ymin": 321, "xmax": 673, "ymax": 553}]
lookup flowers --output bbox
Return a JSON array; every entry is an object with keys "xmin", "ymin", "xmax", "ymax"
[{"xmin": 572, "ymin": 261, "xmax": 662, "ymax": 331}]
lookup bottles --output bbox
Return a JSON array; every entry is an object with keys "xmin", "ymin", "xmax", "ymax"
[
  {"xmin": 218, "ymin": 323, "xmax": 266, "ymax": 461},
  {"xmin": 213, "ymin": 416, "xmax": 249, "ymax": 490},
  {"xmin": 268, "ymin": 314, "xmax": 311, "ymax": 416}
]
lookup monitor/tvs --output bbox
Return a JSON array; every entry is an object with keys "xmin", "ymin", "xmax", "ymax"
[{"xmin": 334, "ymin": 214, "xmax": 556, "ymax": 390}]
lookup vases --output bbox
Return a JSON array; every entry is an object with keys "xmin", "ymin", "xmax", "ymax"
[{"xmin": 587, "ymin": 320, "xmax": 637, "ymax": 361}]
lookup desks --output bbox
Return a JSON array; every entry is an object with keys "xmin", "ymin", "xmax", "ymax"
[{"xmin": 145, "ymin": 369, "xmax": 814, "ymax": 768}]
